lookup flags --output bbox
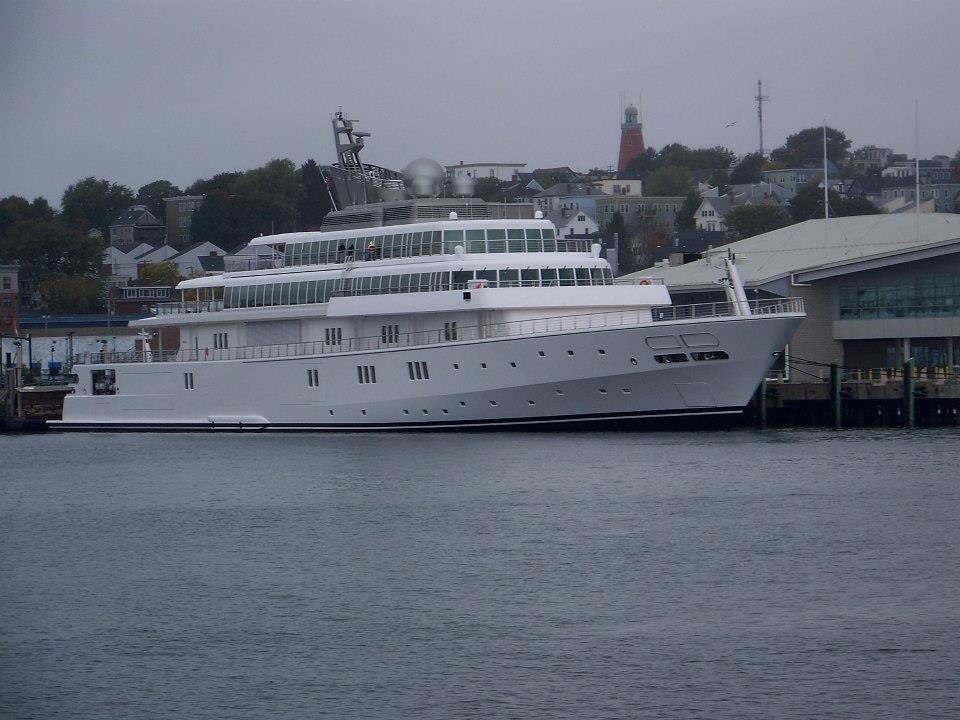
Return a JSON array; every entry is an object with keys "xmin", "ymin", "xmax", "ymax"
[{"xmin": 325, "ymin": 177, "xmax": 333, "ymax": 191}]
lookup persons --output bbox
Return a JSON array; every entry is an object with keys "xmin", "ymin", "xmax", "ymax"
[
  {"xmin": 371, "ymin": 167, "xmax": 386, "ymax": 179},
  {"xmin": 337, "ymin": 243, "xmax": 345, "ymax": 263},
  {"xmin": 347, "ymin": 244, "xmax": 354, "ymax": 261},
  {"xmin": 368, "ymin": 241, "xmax": 375, "ymax": 261}
]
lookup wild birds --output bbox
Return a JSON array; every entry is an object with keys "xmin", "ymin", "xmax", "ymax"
[{"xmin": 724, "ymin": 121, "xmax": 737, "ymax": 128}]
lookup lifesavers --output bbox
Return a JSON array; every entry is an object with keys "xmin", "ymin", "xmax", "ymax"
[{"xmin": 639, "ymin": 280, "xmax": 649, "ymax": 285}]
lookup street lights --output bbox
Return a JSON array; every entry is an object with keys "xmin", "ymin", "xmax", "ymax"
[{"xmin": 1, "ymin": 332, "xmax": 33, "ymax": 381}]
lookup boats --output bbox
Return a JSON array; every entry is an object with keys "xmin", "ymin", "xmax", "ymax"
[{"xmin": 46, "ymin": 102, "xmax": 809, "ymax": 433}]
